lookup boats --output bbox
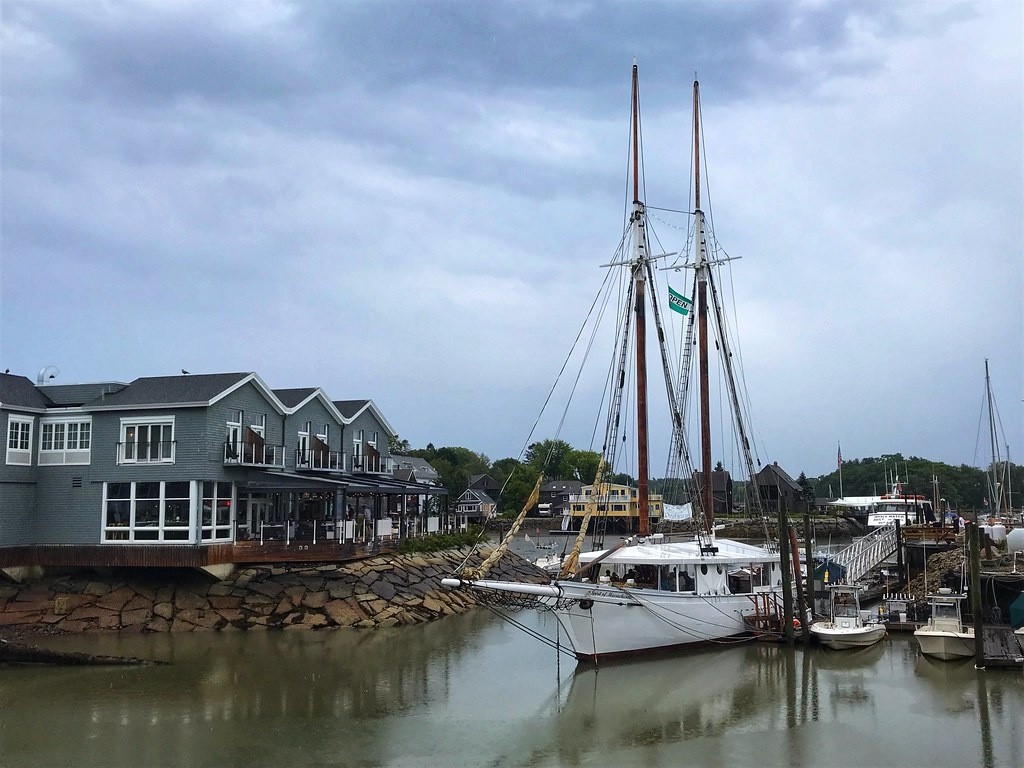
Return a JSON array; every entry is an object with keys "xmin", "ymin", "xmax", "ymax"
[
  {"xmin": 809, "ymin": 581, "xmax": 889, "ymax": 652},
  {"xmin": 913, "ymin": 532, "xmax": 976, "ymax": 662}
]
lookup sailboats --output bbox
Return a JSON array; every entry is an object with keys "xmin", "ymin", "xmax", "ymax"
[
  {"xmin": 826, "ymin": 357, "xmax": 1024, "ymax": 530},
  {"xmin": 440, "ymin": 50, "xmax": 838, "ymax": 666}
]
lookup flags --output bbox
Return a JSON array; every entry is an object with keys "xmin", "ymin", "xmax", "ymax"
[{"xmin": 668, "ymin": 285, "xmax": 692, "ymax": 315}]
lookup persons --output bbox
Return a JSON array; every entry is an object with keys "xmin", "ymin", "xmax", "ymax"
[
  {"xmin": 623, "ymin": 565, "xmax": 641, "ymax": 582},
  {"xmin": 362, "ymin": 506, "xmax": 371, "ymax": 537},
  {"xmin": 952, "ymin": 512, "xmax": 965, "ymax": 534},
  {"xmin": 606, "ymin": 570, "xmax": 619, "ymax": 582},
  {"xmin": 661, "ymin": 568, "xmax": 692, "ymax": 591},
  {"xmin": 348, "ymin": 504, "xmax": 354, "ymax": 520}
]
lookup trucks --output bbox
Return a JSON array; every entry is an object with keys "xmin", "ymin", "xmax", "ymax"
[{"xmin": 538, "ymin": 503, "xmax": 554, "ymax": 518}]
